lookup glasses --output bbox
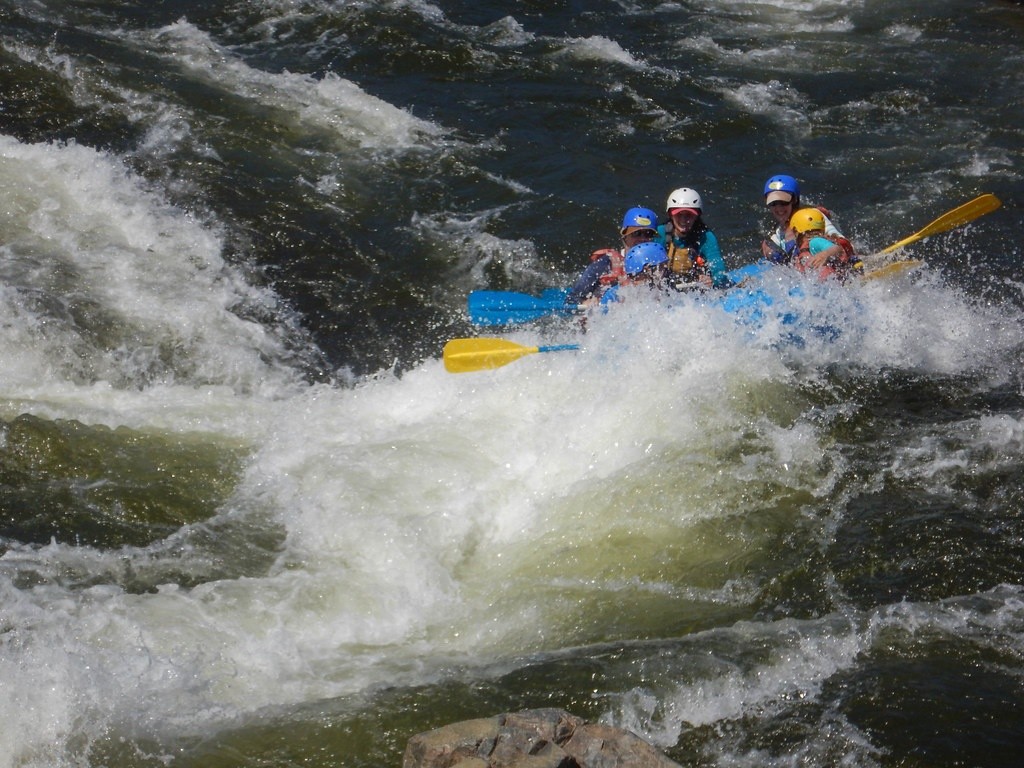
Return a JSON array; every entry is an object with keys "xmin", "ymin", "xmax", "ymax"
[
  {"xmin": 771, "ymin": 201, "xmax": 791, "ymax": 208},
  {"xmin": 633, "ymin": 230, "xmax": 655, "ymax": 239}
]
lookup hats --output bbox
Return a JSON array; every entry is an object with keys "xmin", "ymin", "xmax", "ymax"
[
  {"xmin": 621, "ymin": 225, "xmax": 659, "ymax": 238},
  {"xmin": 766, "ymin": 191, "xmax": 792, "ymax": 206},
  {"xmin": 670, "ymin": 207, "xmax": 699, "ymax": 216}
]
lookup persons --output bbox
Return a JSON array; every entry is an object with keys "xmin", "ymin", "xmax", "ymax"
[{"xmin": 564, "ymin": 173, "xmax": 863, "ymax": 315}]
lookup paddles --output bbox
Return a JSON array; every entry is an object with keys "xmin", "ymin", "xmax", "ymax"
[
  {"xmin": 441, "ymin": 337, "xmax": 584, "ymax": 377},
  {"xmin": 837, "ymin": 258, "xmax": 925, "ymax": 293},
  {"xmin": 849, "ymin": 192, "xmax": 1004, "ymax": 270},
  {"xmin": 537, "ymin": 283, "xmax": 578, "ymax": 307},
  {"xmin": 465, "ymin": 288, "xmax": 592, "ymax": 329}
]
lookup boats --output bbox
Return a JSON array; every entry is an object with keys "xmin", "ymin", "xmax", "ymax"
[{"xmin": 679, "ymin": 260, "xmax": 862, "ymax": 358}]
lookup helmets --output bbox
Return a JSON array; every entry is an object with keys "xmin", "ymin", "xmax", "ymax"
[
  {"xmin": 763, "ymin": 174, "xmax": 800, "ymax": 198},
  {"xmin": 625, "ymin": 242, "xmax": 670, "ymax": 278},
  {"xmin": 619, "ymin": 205, "xmax": 658, "ymax": 232},
  {"xmin": 790, "ymin": 208, "xmax": 825, "ymax": 234},
  {"xmin": 666, "ymin": 187, "xmax": 703, "ymax": 215}
]
